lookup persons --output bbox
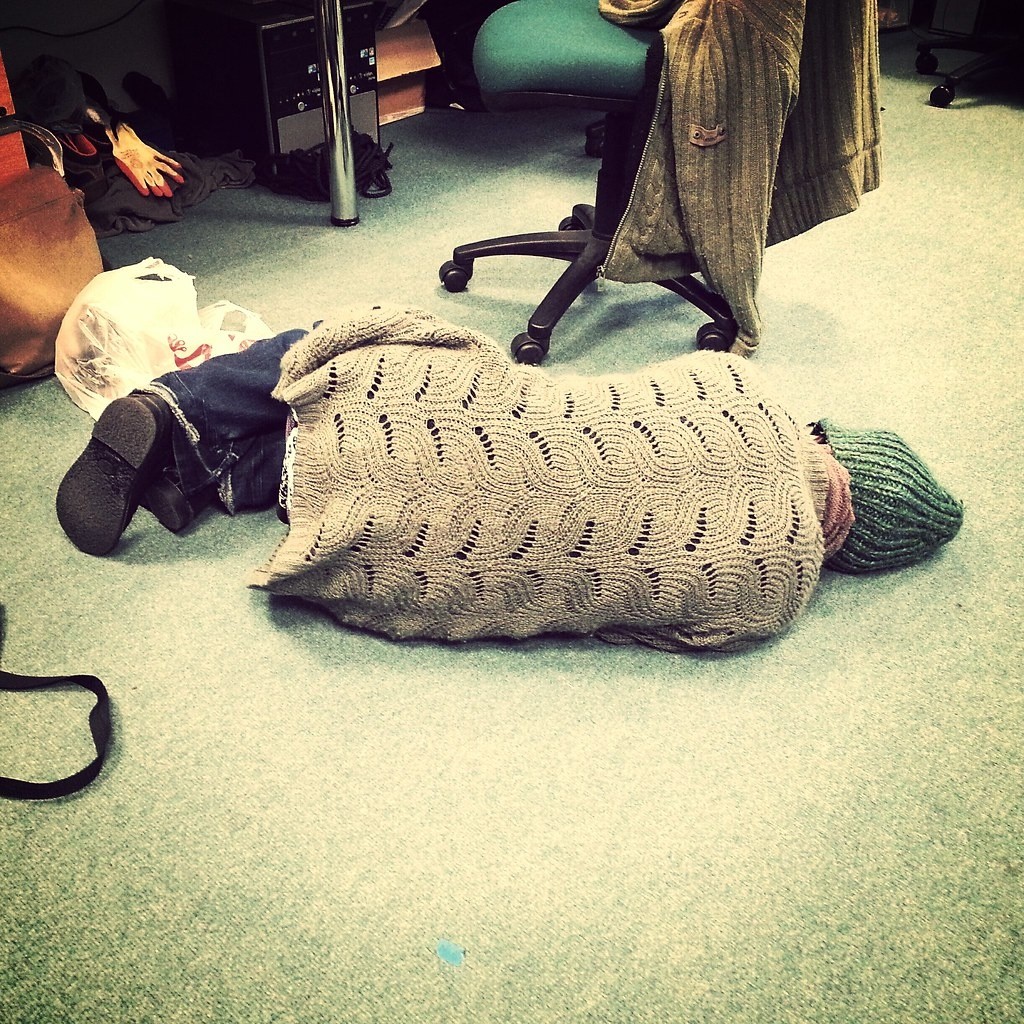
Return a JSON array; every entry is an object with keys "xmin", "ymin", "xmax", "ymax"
[{"xmin": 53, "ymin": 310, "xmax": 962, "ymax": 655}]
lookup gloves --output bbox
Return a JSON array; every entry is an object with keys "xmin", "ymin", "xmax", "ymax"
[{"xmin": 106, "ymin": 99, "xmax": 184, "ymax": 198}]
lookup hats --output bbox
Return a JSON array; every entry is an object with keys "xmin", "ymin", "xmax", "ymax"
[{"xmin": 809, "ymin": 416, "xmax": 965, "ymax": 575}]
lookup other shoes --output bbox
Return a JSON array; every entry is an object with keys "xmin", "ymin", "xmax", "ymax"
[
  {"xmin": 54, "ymin": 387, "xmax": 173, "ymax": 559},
  {"xmin": 138, "ymin": 457, "xmax": 197, "ymax": 535}
]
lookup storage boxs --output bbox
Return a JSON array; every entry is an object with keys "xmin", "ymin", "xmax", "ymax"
[{"xmin": 376, "ymin": 19, "xmax": 443, "ymax": 125}]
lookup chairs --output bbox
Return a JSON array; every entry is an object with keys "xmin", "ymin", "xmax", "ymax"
[
  {"xmin": 916, "ymin": 35, "xmax": 1024, "ymax": 106},
  {"xmin": 440, "ymin": 0, "xmax": 885, "ymax": 370}
]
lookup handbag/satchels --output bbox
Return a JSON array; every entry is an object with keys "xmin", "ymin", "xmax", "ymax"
[
  {"xmin": 0, "ymin": 123, "xmax": 104, "ymax": 387},
  {"xmin": 54, "ymin": 256, "xmax": 201, "ymax": 420},
  {"xmin": 196, "ymin": 300, "xmax": 279, "ymax": 358}
]
